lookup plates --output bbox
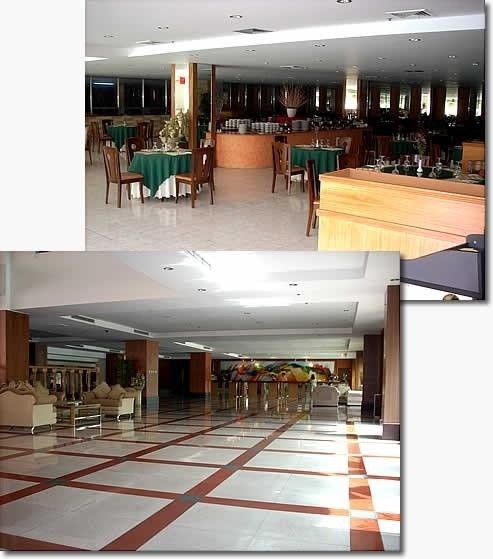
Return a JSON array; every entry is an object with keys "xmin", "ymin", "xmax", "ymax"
[{"xmin": 290, "ymin": 121, "xmax": 309, "ymax": 131}]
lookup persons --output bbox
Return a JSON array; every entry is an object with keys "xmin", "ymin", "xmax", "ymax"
[
  {"xmin": 279, "ymin": 373, "xmax": 287, "ymax": 381},
  {"xmin": 309, "ymin": 374, "xmax": 317, "ymax": 392}
]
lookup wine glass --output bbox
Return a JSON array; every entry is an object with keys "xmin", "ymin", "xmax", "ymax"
[
  {"xmin": 403, "ymin": 155, "xmax": 411, "ymax": 167},
  {"xmin": 150, "ymin": 141, "xmax": 183, "ymax": 155},
  {"xmin": 379, "ymin": 156, "xmax": 386, "ymax": 170},
  {"xmin": 391, "ymin": 163, "xmax": 400, "ymax": 175},
  {"xmin": 391, "ymin": 132, "xmax": 417, "ymax": 141},
  {"xmin": 435, "ymin": 157, "xmax": 443, "ymax": 170},
  {"xmin": 454, "ymin": 161, "xmax": 463, "ymax": 176},
  {"xmin": 373, "ymin": 158, "xmax": 381, "ymax": 174}
]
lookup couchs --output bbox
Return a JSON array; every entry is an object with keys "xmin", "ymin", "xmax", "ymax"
[
  {"xmin": 311, "ymin": 382, "xmax": 363, "ymax": 407},
  {"xmin": 0, "ymin": 379, "xmax": 136, "ymax": 437}
]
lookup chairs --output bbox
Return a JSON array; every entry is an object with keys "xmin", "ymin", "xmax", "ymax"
[
  {"xmin": 85, "ymin": 117, "xmax": 218, "ymax": 206},
  {"xmin": 269, "ymin": 111, "xmax": 485, "ymax": 238}
]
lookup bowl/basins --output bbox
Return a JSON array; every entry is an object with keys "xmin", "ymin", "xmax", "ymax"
[{"xmin": 224, "ymin": 119, "xmax": 279, "ymax": 131}]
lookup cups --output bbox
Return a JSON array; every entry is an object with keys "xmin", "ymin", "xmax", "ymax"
[{"xmin": 310, "ymin": 139, "xmax": 332, "ymax": 148}]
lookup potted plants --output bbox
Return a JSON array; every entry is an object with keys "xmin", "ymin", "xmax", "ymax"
[{"xmin": 275, "ymin": 80, "xmax": 310, "ymax": 117}]
